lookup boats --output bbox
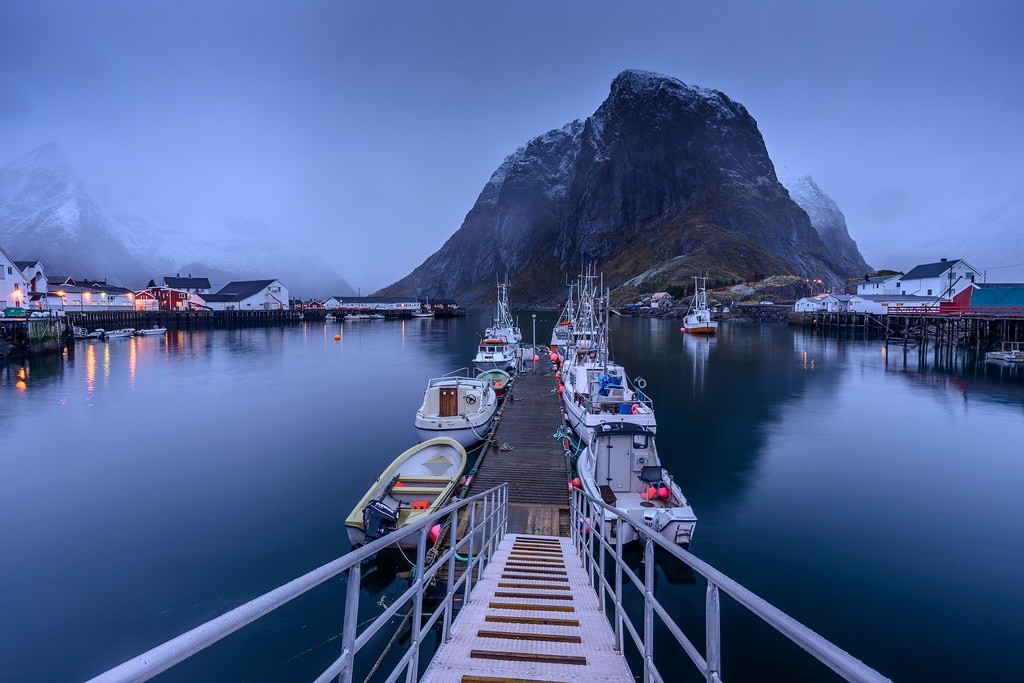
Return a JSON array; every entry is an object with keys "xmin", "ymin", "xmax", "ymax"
[
  {"xmin": 413, "ymin": 367, "xmax": 498, "ymax": 448},
  {"xmin": 679, "ymin": 273, "xmax": 718, "ymax": 333},
  {"xmin": 549, "ymin": 260, "xmax": 699, "ymax": 544},
  {"xmin": 344, "ymin": 437, "xmax": 467, "ymax": 549},
  {"xmin": 89, "ymin": 325, "xmax": 168, "ymax": 338},
  {"xmin": 985, "ymin": 342, "xmax": 1024, "ymax": 361},
  {"xmin": 683, "ymin": 333, "xmax": 718, "ymax": 400},
  {"xmin": 324, "ymin": 287, "xmax": 385, "ymax": 320},
  {"xmin": 472, "ymin": 272, "xmax": 522, "ymax": 372},
  {"xmin": 476, "ymin": 369, "xmax": 510, "ymax": 396},
  {"xmin": 410, "ymin": 296, "xmax": 436, "ymax": 317},
  {"xmin": 984, "ymin": 359, "xmax": 1024, "ymax": 384}
]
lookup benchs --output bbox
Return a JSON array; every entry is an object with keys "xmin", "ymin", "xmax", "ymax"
[
  {"xmin": 390, "ymin": 487, "xmax": 445, "ymax": 503},
  {"xmin": 398, "ymin": 475, "xmax": 452, "ymax": 487}
]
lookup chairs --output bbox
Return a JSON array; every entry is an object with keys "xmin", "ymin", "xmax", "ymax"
[{"xmin": 637, "ymin": 465, "xmax": 663, "ymax": 508}]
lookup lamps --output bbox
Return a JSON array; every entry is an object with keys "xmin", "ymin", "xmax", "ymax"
[{"xmin": 602, "ymin": 425, "xmax": 612, "ymax": 432}]
